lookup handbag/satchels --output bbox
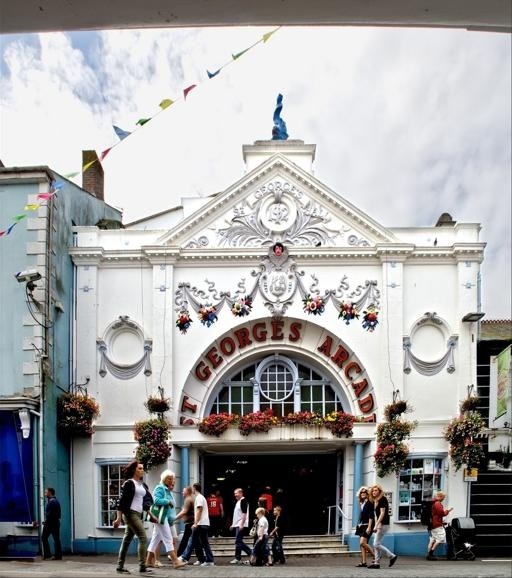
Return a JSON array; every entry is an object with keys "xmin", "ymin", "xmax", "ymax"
[
  {"xmin": 144, "ymin": 504, "xmax": 168, "ymax": 525},
  {"xmin": 249, "ymin": 527, "xmax": 256, "ymax": 538}
]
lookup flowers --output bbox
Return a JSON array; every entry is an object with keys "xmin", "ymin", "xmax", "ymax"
[
  {"xmin": 238, "ymin": 406, "xmax": 278, "ymax": 435},
  {"xmin": 323, "ymin": 410, "xmax": 355, "ymax": 438},
  {"xmin": 176, "ymin": 294, "xmax": 379, "ymax": 334},
  {"xmin": 456, "ymin": 388, "xmax": 479, "ymax": 410},
  {"xmin": 283, "ymin": 410, "xmax": 321, "ymax": 426},
  {"xmin": 59, "ymin": 393, "xmax": 101, "ymax": 435},
  {"xmin": 197, "ymin": 412, "xmax": 233, "ymax": 436},
  {"xmin": 374, "ymin": 421, "xmax": 415, "ymax": 478},
  {"xmin": 134, "ymin": 419, "xmax": 172, "ymax": 470},
  {"xmin": 445, "ymin": 409, "xmax": 485, "ymax": 472},
  {"xmin": 140, "ymin": 395, "xmax": 171, "ymax": 414},
  {"xmin": 383, "ymin": 400, "xmax": 415, "ymax": 416}
]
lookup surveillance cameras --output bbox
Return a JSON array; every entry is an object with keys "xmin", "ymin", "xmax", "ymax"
[
  {"xmin": 295, "ymin": 378, "xmax": 303, "ymax": 386},
  {"xmin": 251, "ymin": 378, "xmax": 259, "ymax": 386},
  {"xmin": 13, "ymin": 270, "xmax": 42, "ymax": 283}
]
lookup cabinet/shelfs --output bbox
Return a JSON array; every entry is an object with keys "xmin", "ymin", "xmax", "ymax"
[
  {"xmin": 99, "ymin": 463, "xmax": 134, "ymax": 526},
  {"xmin": 399, "ymin": 456, "xmax": 442, "ymax": 521}
]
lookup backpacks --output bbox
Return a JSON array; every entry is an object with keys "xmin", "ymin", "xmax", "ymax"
[{"xmin": 420, "ymin": 500, "xmax": 437, "ymax": 530}]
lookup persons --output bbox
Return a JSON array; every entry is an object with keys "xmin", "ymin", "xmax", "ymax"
[
  {"xmin": 228, "ymin": 488, "xmax": 250, "ymax": 564},
  {"xmin": 425, "ymin": 491, "xmax": 453, "ymax": 561},
  {"xmin": 176, "ymin": 482, "xmax": 207, "ymax": 565},
  {"xmin": 355, "ymin": 485, "xmax": 373, "ymax": 569},
  {"xmin": 269, "ymin": 506, "xmax": 287, "ymax": 565},
  {"xmin": 41, "ymin": 487, "xmax": 63, "ymax": 560},
  {"xmin": 113, "ymin": 460, "xmax": 157, "ymax": 574},
  {"xmin": 258, "ymin": 478, "xmax": 283, "ymax": 514},
  {"xmin": 206, "ymin": 488, "xmax": 225, "ymax": 538},
  {"xmin": 179, "ymin": 484, "xmax": 214, "ymax": 567},
  {"xmin": 249, "ymin": 506, "xmax": 274, "ymax": 566},
  {"xmin": 144, "ymin": 469, "xmax": 188, "ymax": 570},
  {"xmin": 367, "ymin": 483, "xmax": 397, "ymax": 569},
  {"xmin": 156, "ymin": 478, "xmax": 177, "ymax": 568}
]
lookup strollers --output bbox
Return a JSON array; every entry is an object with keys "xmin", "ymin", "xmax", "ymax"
[{"xmin": 443, "ymin": 515, "xmax": 478, "ymax": 561}]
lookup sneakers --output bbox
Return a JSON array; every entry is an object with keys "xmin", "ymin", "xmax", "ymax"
[
  {"xmin": 356, "ymin": 556, "xmax": 437, "ymax": 569},
  {"xmin": 116, "ymin": 555, "xmax": 273, "ymax": 574}
]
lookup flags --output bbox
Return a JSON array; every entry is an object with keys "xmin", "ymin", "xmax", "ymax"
[{"xmin": 494, "ymin": 344, "xmax": 510, "ymax": 418}]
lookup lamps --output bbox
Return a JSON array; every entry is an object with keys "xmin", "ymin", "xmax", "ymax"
[{"xmin": 461, "ymin": 309, "xmax": 485, "ymax": 324}]
